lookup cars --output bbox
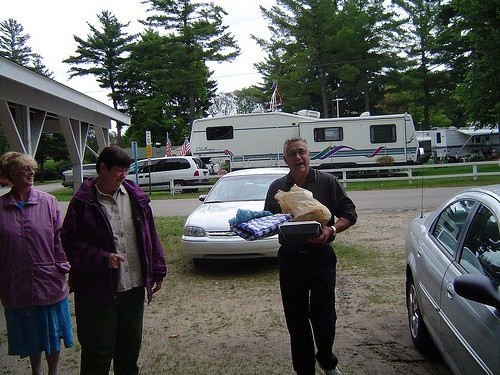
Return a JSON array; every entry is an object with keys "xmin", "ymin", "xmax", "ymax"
[
  {"xmin": 404, "ymin": 183, "xmax": 500, "ymax": 375},
  {"xmin": 180, "ymin": 168, "xmax": 290, "ymax": 265},
  {"xmin": 61, "ymin": 164, "xmax": 99, "ymax": 188}
]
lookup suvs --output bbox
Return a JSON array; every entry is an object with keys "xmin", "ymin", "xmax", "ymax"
[{"xmin": 123, "ymin": 156, "xmax": 210, "ymax": 193}]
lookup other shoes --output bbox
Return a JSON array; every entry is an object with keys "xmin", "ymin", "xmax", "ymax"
[{"xmin": 319, "ymin": 363, "xmax": 342, "ymax": 375}]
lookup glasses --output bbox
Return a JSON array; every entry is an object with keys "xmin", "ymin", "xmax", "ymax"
[
  {"xmin": 23, "ymin": 166, "xmax": 38, "ymax": 173},
  {"xmin": 286, "ymin": 147, "xmax": 308, "ymax": 158}
]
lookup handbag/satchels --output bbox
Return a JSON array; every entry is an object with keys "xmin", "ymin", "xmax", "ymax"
[{"xmin": 274, "ymin": 182, "xmax": 332, "ymax": 226}]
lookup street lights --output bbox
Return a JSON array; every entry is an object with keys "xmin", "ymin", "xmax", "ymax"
[{"xmin": 332, "ymin": 98, "xmax": 343, "ymax": 118}]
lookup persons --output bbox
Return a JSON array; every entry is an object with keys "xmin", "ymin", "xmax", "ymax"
[
  {"xmin": 487, "ymin": 147, "xmax": 492, "ymax": 154},
  {"xmin": 263, "ymin": 136, "xmax": 357, "ymax": 375},
  {"xmin": 442, "ymin": 150, "xmax": 446, "ymax": 160},
  {"xmin": 475, "ymin": 149, "xmax": 484, "ymax": 155},
  {"xmin": 225, "ymin": 149, "xmax": 232, "ymax": 172},
  {"xmin": 0, "ymin": 152, "xmax": 74, "ymax": 375},
  {"xmin": 61, "ymin": 146, "xmax": 167, "ymax": 375}
]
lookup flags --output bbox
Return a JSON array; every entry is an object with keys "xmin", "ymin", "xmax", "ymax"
[
  {"xmin": 165, "ymin": 139, "xmax": 171, "ymax": 156},
  {"xmin": 181, "ymin": 140, "xmax": 191, "ymax": 156}
]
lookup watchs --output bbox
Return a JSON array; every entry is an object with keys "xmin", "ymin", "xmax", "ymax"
[{"xmin": 331, "ymin": 226, "xmax": 337, "ymax": 236}]
